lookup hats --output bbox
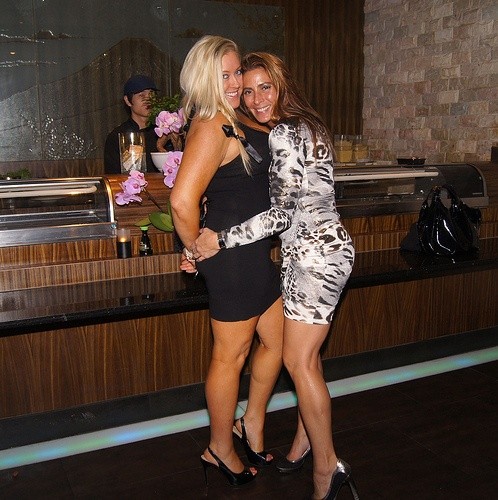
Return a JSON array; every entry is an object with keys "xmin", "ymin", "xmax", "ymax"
[{"xmin": 123, "ymin": 74, "xmax": 159, "ymax": 95}]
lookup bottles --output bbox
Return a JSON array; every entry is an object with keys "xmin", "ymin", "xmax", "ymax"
[
  {"xmin": 139, "ymin": 226, "xmax": 152, "ymax": 257},
  {"xmin": 491, "ymin": 142, "xmax": 498, "ymax": 162}
]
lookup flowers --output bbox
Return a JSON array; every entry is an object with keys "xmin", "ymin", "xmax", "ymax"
[{"xmin": 115, "ymin": 88, "xmax": 188, "ymax": 232}]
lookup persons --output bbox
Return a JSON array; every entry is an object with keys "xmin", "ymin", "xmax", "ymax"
[
  {"xmin": 103, "ymin": 75, "xmax": 187, "ymax": 176},
  {"xmin": 168, "ymin": 34, "xmax": 285, "ymax": 487},
  {"xmin": 189, "ymin": 53, "xmax": 362, "ymax": 500}
]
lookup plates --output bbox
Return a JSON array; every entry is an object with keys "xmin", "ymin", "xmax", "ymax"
[{"xmin": 396, "ymin": 158, "xmax": 427, "ymax": 165}]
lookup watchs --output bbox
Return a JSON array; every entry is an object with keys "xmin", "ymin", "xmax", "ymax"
[{"xmin": 217, "ymin": 232, "xmax": 226, "ymax": 247}]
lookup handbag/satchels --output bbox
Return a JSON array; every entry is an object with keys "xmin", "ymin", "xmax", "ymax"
[{"xmin": 417, "ymin": 186, "xmax": 482, "ymax": 260}]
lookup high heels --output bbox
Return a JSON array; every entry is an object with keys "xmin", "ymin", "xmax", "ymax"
[
  {"xmin": 199, "ymin": 443, "xmax": 259, "ymax": 486},
  {"xmin": 310, "ymin": 457, "xmax": 357, "ymax": 500},
  {"xmin": 274, "ymin": 446, "xmax": 311, "ymax": 470},
  {"xmin": 231, "ymin": 416, "xmax": 273, "ymax": 466}
]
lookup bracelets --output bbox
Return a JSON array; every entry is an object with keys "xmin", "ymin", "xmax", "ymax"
[{"xmin": 186, "ymin": 249, "xmax": 196, "ymax": 262}]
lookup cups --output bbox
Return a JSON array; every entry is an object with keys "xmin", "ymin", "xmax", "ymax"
[
  {"xmin": 116, "ymin": 241, "xmax": 132, "ymax": 259},
  {"xmin": 118, "ymin": 131, "xmax": 147, "ymax": 174},
  {"xmin": 351, "ymin": 134, "xmax": 370, "ymax": 162},
  {"xmin": 332, "ymin": 134, "xmax": 353, "ymax": 163}
]
